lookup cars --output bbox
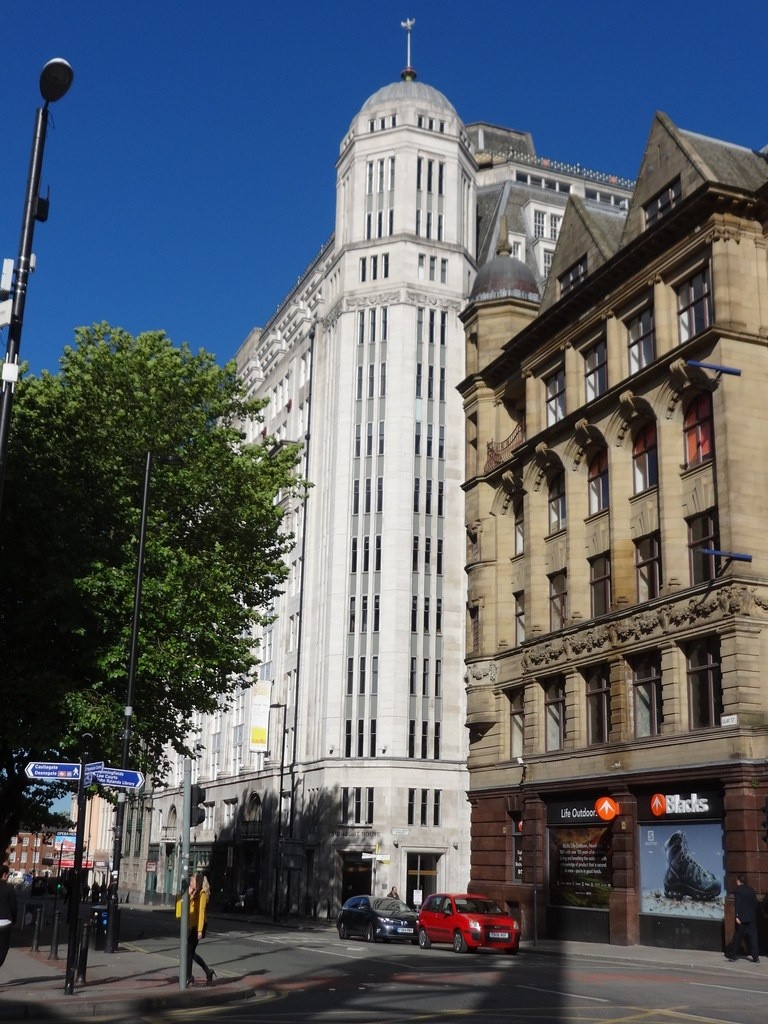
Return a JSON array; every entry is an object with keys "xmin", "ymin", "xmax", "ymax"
[{"xmin": 336, "ymin": 894, "xmax": 420, "ymax": 945}]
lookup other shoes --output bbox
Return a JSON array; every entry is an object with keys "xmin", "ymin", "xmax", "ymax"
[
  {"xmin": 725, "ymin": 953, "xmax": 735, "ymax": 962},
  {"xmin": 754, "ymin": 960, "xmax": 761, "ymax": 963}
]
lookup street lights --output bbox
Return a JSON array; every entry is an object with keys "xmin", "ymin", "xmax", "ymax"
[{"xmin": 0, "ymin": 58, "xmax": 75, "ymax": 447}]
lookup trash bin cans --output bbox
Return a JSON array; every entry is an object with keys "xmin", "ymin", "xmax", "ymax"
[
  {"xmin": 20, "ymin": 903, "xmax": 45, "ymax": 932},
  {"xmin": 90, "ymin": 904, "xmax": 123, "ymax": 951}
]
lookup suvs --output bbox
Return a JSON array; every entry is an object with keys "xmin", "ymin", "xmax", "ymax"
[{"xmin": 418, "ymin": 893, "xmax": 522, "ymax": 955}]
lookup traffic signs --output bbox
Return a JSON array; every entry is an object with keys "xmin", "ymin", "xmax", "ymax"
[{"xmin": 25, "ymin": 760, "xmax": 146, "ymax": 790}]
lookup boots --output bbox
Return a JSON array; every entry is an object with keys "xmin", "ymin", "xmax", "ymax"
[{"xmin": 663, "ymin": 831, "xmax": 722, "ymax": 900}]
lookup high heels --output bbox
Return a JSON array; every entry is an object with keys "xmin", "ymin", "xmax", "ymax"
[
  {"xmin": 206, "ymin": 969, "xmax": 217, "ymax": 986},
  {"xmin": 186, "ymin": 976, "xmax": 195, "ymax": 987}
]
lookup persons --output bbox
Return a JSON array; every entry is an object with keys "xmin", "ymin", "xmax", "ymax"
[
  {"xmin": 177, "ymin": 873, "xmax": 217, "ymax": 986},
  {"xmin": 724, "ymin": 874, "xmax": 760, "ymax": 962},
  {"xmin": 64, "ymin": 881, "xmax": 110, "ymax": 905},
  {"xmin": 0, "ymin": 865, "xmax": 17, "ymax": 967},
  {"xmin": 388, "ymin": 887, "xmax": 400, "ymax": 898}
]
